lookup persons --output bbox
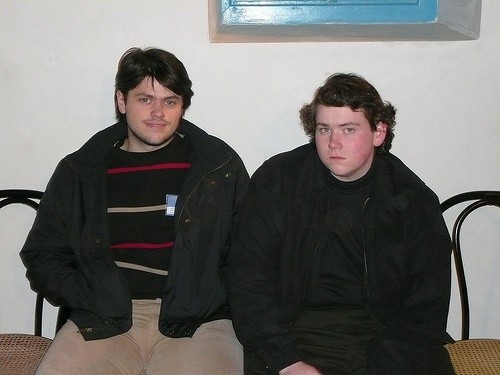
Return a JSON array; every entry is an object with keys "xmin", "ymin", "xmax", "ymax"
[
  {"xmin": 19, "ymin": 46, "xmax": 251, "ymax": 375},
  {"xmin": 229, "ymin": 74, "xmax": 451, "ymax": 375}
]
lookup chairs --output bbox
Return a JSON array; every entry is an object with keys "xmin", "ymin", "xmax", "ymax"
[
  {"xmin": 437, "ymin": 190, "xmax": 500, "ymax": 375},
  {"xmin": 0, "ymin": 188, "xmax": 56, "ymax": 375}
]
